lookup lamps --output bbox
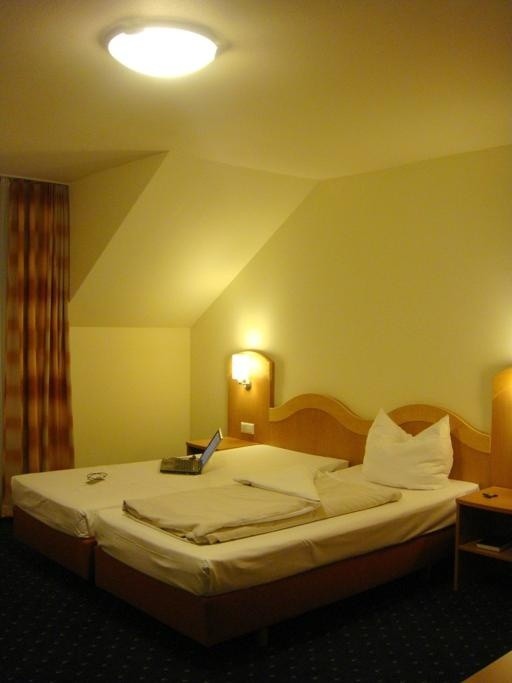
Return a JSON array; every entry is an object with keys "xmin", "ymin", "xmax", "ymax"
[{"xmin": 98, "ymin": 21, "xmax": 221, "ymax": 83}]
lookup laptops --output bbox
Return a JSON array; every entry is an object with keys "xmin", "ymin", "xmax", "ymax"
[{"xmin": 159, "ymin": 427, "xmax": 223, "ymax": 475}]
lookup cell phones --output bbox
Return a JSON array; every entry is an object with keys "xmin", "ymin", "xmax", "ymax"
[{"xmin": 482, "ymin": 491, "xmax": 498, "ymax": 497}]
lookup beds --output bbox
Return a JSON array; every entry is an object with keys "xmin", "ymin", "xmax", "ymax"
[
  {"xmin": 11, "ymin": 442, "xmax": 349, "ymax": 576},
  {"xmin": 95, "ymin": 462, "xmax": 480, "ymax": 643}
]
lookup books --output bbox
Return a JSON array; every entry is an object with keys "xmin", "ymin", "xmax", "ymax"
[{"xmin": 475, "ymin": 533, "xmax": 510, "ymax": 552}]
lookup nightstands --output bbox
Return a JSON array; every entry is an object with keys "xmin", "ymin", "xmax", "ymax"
[
  {"xmin": 455, "ymin": 483, "xmax": 512, "ymax": 583},
  {"xmin": 186, "ymin": 436, "xmax": 258, "ymax": 456}
]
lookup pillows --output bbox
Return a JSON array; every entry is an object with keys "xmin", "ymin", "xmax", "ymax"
[{"xmin": 360, "ymin": 410, "xmax": 454, "ymax": 486}]
